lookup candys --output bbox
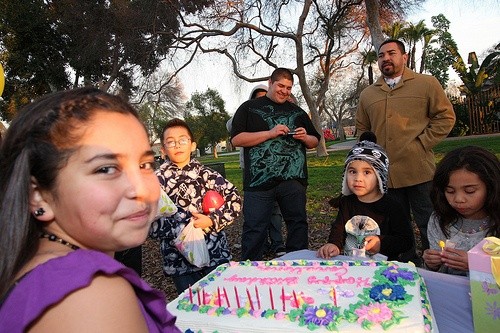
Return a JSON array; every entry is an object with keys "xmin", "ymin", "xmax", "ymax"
[{"xmin": 439, "ymin": 240, "xmax": 445, "ymax": 252}]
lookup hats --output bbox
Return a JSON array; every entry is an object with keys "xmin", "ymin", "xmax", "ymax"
[{"xmin": 341, "ymin": 131, "xmax": 389, "ymax": 196}]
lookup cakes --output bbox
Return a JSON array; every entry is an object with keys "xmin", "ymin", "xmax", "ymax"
[{"xmin": 166, "ymin": 259, "xmax": 433, "ymax": 333}]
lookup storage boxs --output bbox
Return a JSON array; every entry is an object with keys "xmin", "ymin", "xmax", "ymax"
[{"xmin": 467, "ymin": 239, "xmax": 500, "ymax": 333}]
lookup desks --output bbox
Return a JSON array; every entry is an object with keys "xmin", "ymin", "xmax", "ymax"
[{"xmin": 272, "ymin": 249, "xmax": 474, "ymax": 333}]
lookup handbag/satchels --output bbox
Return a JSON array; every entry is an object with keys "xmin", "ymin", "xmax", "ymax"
[
  {"xmin": 154, "ymin": 188, "xmax": 178, "ymax": 220},
  {"xmin": 173, "ymin": 219, "xmax": 210, "ymax": 268}
]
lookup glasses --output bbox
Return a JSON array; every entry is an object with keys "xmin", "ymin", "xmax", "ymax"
[{"xmin": 163, "ymin": 138, "xmax": 191, "ymax": 148}]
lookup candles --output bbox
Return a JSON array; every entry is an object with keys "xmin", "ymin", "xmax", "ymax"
[
  {"xmin": 202, "ymin": 287, "xmax": 206, "ymax": 305},
  {"xmin": 282, "ymin": 286, "xmax": 286, "ymax": 311},
  {"xmin": 268, "ymin": 287, "xmax": 274, "ymax": 310},
  {"xmin": 217, "ymin": 286, "xmax": 221, "ymax": 307},
  {"xmin": 246, "ymin": 287, "xmax": 254, "ymax": 310},
  {"xmin": 234, "ymin": 285, "xmax": 240, "ymax": 307},
  {"xmin": 292, "ymin": 289, "xmax": 299, "ymax": 308},
  {"xmin": 255, "ymin": 285, "xmax": 261, "ymax": 309},
  {"xmin": 197, "ymin": 287, "xmax": 201, "ymax": 305},
  {"xmin": 189, "ymin": 284, "xmax": 193, "ymax": 303},
  {"xmin": 223, "ymin": 286, "xmax": 231, "ymax": 307},
  {"xmin": 333, "ymin": 288, "xmax": 337, "ymax": 305}
]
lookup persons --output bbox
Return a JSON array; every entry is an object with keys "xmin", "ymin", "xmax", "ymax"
[
  {"xmin": 421, "ymin": 146, "xmax": 500, "ymax": 277},
  {"xmin": 148, "ymin": 118, "xmax": 242, "ymax": 294},
  {"xmin": 226, "ymin": 68, "xmax": 322, "ymax": 262},
  {"xmin": 318, "ymin": 140, "xmax": 414, "ymax": 260},
  {"xmin": 0, "ymin": 87, "xmax": 178, "ymax": 333},
  {"xmin": 356, "ymin": 39, "xmax": 456, "ymax": 269}
]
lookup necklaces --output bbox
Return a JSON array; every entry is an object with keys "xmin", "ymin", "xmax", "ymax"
[{"xmin": 37, "ymin": 231, "xmax": 82, "ymax": 251}]
